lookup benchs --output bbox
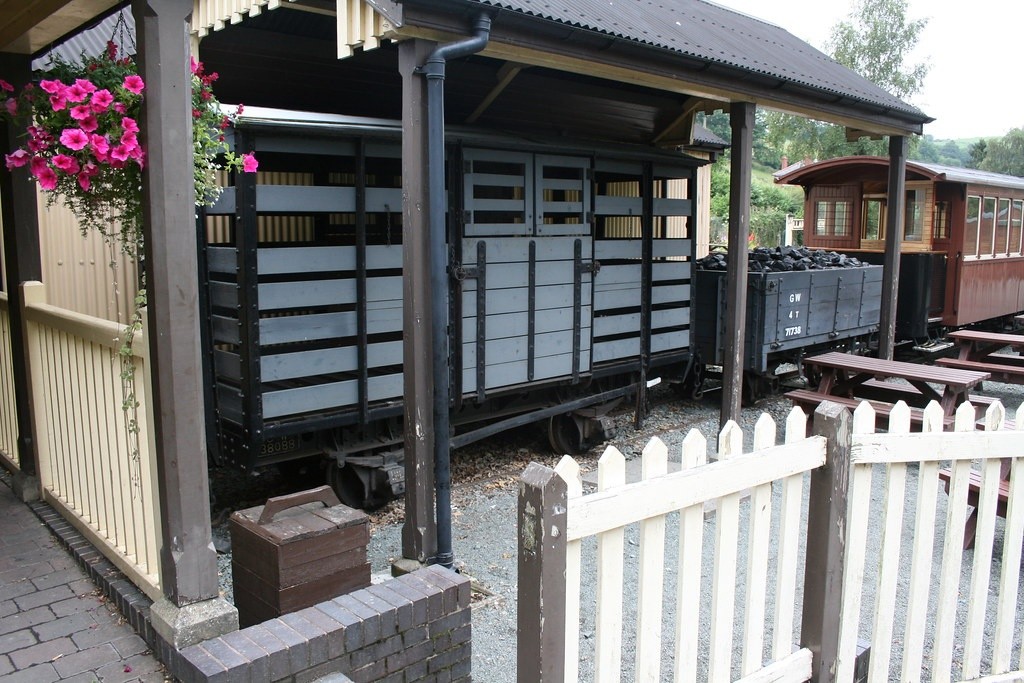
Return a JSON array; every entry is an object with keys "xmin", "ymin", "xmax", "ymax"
[{"xmin": 784, "ymin": 353, "xmax": 1024, "ymax": 553}]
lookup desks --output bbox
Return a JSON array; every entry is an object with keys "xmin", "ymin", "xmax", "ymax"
[
  {"xmin": 802, "ymin": 353, "xmax": 992, "ymax": 438},
  {"xmin": 945, "ymin": 328, "xmax": 1024, "ymax": 365}
]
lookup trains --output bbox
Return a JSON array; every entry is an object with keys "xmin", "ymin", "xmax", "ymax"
[{"xmin": 665, "ymin": 156, "xmax": 1024, "ymax": 410}]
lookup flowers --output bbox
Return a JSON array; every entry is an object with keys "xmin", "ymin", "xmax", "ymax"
[{"xmin": 0, "ymin": 40, "xmax": 259, "ymax": 456}]
